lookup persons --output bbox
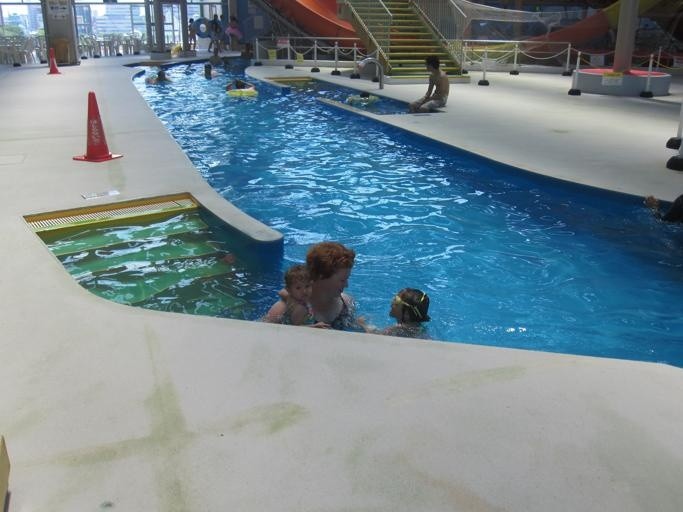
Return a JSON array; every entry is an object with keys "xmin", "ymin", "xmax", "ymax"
[
  {"xmin": 143, "ymin": 71, "xmax": 172, "ymax": 84},
  {"xmin": 237, "ymin": 42, "xmax": 254, "ymax": 59},
  {"xmin": 344, "ymin": 93, "xmax": 379, "ymax": 107},
  {"xmin": 225, "ymin": 17, "xmax": 242, "ymax": 43},
  {"xmin": 258, "ymin": 241, "xmax": 377, "ymax": 334},
  {"xmin": 207, "ymin": 48, "xmax": 222, "ymax": 66},
  {"xmin": 218, "ymin": 15, "xmax": 229, "ymax": 52},
  {"xmin": 226, "ymin": 78, "xmax": 254, "ymax": 93},
  {"xmin": 199, "ymin": 63, "xmax": 217, "ymax": 75},
  {"xmin": 406, "ymin": 55, "xmax": 449, "ymax": 114},
  {"xmin": 277, "ymin": 264, "xmax": 333, "ymax": 328},
  {"xmin": 207, "ymin": 14, "xmax": 223, "ymax": 53},
  {"xmin": 378, "ymin": 286, "xmax": 432, "ymax": 340},
  {"xmin": 643, "ymin": 189, "xmax": 682, "ymax": 226},
  {"xmin": 188, "ymin": 18, "xmax": 196, "ymax": 51}
]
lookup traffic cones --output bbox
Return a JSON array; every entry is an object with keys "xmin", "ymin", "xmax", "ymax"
[
  {"xmin": 73, "ymin": 92, "xmax": 122, "ymax": 163},
  {"xmin": 46, "ymin": 48, "xmax": 61, "ymax": 74}
]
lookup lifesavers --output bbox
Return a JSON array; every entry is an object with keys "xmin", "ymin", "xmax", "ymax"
[
  {"xmin": 346, "ymin": 94, "xmax": 379, "ymax": 106},
  {"xmin": 192, "ymin": 17, "xmax": 210, "ymax": 38},
  {"xmin": 208, "ymin": 19, "xmax": 225, "ymax": 41},
  {"xmin": 225, "ymin": 27, "xmax": 242, "ymax": 41},
  {"xmin": 226, "ymin": 89, "xmax": 258, "ymax": 97}
]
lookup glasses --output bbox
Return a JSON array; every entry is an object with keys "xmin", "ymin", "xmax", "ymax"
[{"xmin": 394, "ymin": 295, "xmax": 403, "ymax": 305}]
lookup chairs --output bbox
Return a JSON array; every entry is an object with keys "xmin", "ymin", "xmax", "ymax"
[{"xmin": 0, "ymin": 31, "xmax": 153, "ymax": 66}]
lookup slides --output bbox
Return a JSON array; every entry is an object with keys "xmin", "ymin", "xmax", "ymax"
[
  {"xmin": 463, "ymin": 0, "xmax": 660, "ymax": 61},
  {"xmin": 271, "ymin": 1, "xmax": 371, "ymax": 51}
]
